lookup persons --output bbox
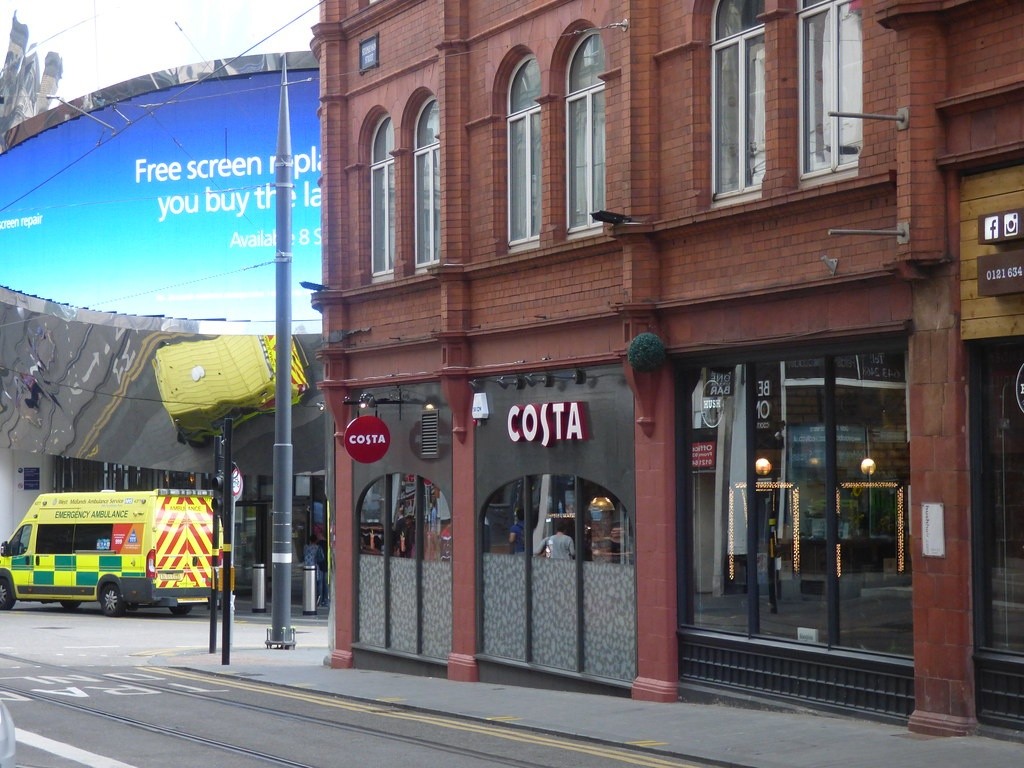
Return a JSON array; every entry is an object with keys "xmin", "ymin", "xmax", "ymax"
[
  {"xmin": 588, "ymin": 523, "xmax": 636, "ymax": 563},
  {"xmin": 509, "ymin": 508, "xmax": 525, "ymax": 556},
  {"xmin": 393, "ymin": 500, "xmax": 452, "ymax": 563},
  {"xmin": 304, "ymin": 530, "xmax": 329, "ymax": 607},
  {"xmin": 545, "ymin": 523, "xmax": 575, "ymax": 560}
]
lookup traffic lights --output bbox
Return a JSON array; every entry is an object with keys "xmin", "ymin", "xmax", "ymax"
[{"xmin": 210, "ymin": 470, "xmax": 225, "ymax": 517}]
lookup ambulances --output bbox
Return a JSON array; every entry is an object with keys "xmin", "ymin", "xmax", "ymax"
[{"xmin": 0, "ymin": 488, "xmax": 226, "ymax": 617}]
